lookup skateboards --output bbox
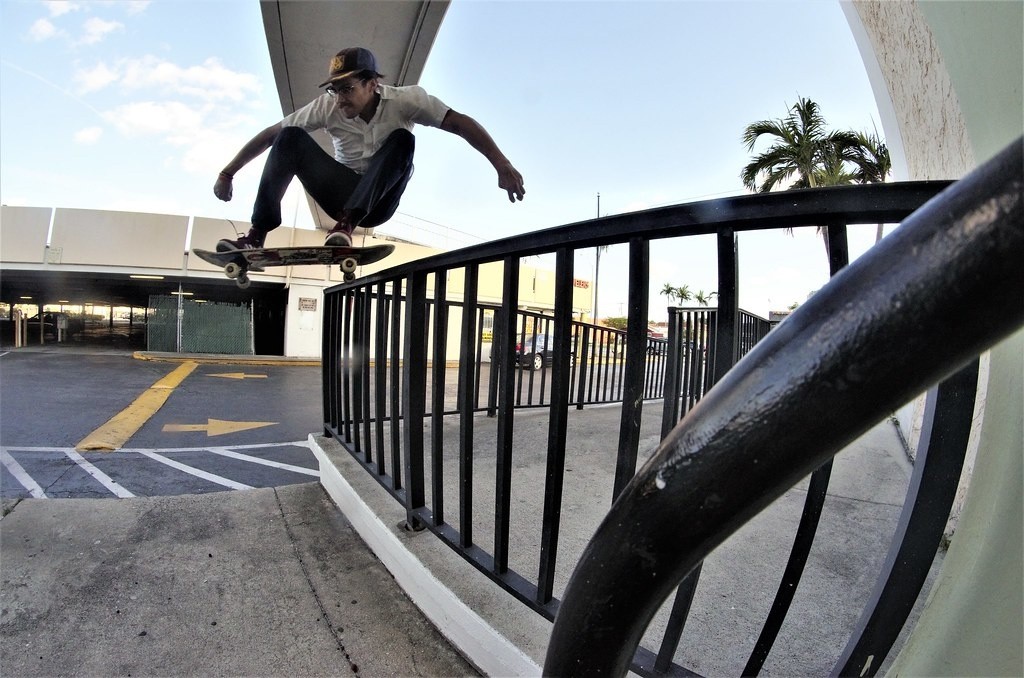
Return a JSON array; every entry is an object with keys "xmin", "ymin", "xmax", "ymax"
[{"xmin": 192, "ymin": 243, "xmax": 396, "ymax": 290}]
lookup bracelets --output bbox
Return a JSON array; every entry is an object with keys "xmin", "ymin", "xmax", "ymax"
[{"xmin": 219, "ymin": 171, "xmax": 233, "ymax": 180}]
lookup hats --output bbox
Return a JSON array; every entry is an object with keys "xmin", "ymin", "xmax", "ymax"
[{"xmin": 318, "ymin": 47, "xmax": 377, "ymax": 88}]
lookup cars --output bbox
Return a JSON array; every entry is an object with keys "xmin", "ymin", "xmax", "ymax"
[
  {"xmin": 489, "ymin": 333, "xmax": 579, "ymax": 371},
  {"xmin": 646, "ymin": 337, "xmax": 691, "ymax": 358}
]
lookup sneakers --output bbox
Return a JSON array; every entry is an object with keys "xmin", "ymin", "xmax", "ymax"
[
  {"xmin": 324, "ymin": 222, "xmax": 353, "ymax": 248},
  {"xmin": 216, "ymin": 237, "xmax": 265, "ymax": 272}
]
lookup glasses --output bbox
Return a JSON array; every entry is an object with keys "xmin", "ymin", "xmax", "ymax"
[{"xmin": 325, "ymin": 78, "xmax": 365, "ymax": 98}]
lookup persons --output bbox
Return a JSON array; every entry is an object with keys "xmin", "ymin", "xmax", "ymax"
[{"xmin": 213, "ymin": 47, "xmax": 526, "ymax": 252}]
